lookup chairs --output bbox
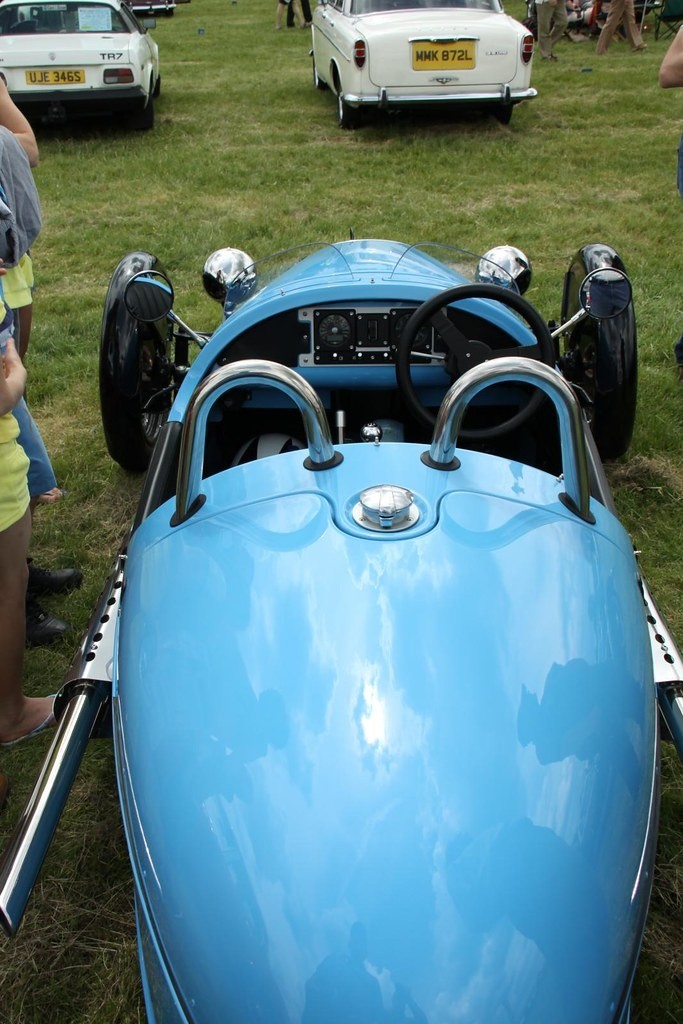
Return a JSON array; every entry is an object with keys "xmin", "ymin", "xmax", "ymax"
[{"xmin": 653, "ymin": 0, "xmax": 683, "ymax": 40}]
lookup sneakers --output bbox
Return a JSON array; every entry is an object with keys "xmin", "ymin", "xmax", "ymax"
[{"xmin": 24, "ymin": 550, "xmax": 83, "ymax": 646}]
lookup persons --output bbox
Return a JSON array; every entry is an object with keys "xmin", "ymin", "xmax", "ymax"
[
  {"xmin": 0, "ymin": 69, "xmax": 85, "ymax": 752},
  {"xmin": 595, "ymin": 0, "xmax": 647, "ymax": 55},
  {"xmin": 534, "ymin": 0, "xmax": 568, "ymax": 63},
  {"xmin": 273, "ymin": 0, "xmax": 312, "ymax": 31},
  {"xmin": 657, "ymin": 20, "xmax": 683, "ymax": 364}
]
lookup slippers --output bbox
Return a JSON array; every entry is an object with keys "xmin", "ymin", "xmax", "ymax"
[{"xmin": 0, "ymin": 692, "xmax": 70, "ymax": 749}]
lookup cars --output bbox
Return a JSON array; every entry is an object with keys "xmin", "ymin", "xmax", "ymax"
[
  {"xmin": 310, "ymin": 0, "xmax": 540, "ymax": 127},
  {"xmin": 0, "ymin": 0, "xmax": 162, "ymax": 131},
  {"xmin": 128, "ymin": 0, "xmax": 177, "ymax": 17}
]
